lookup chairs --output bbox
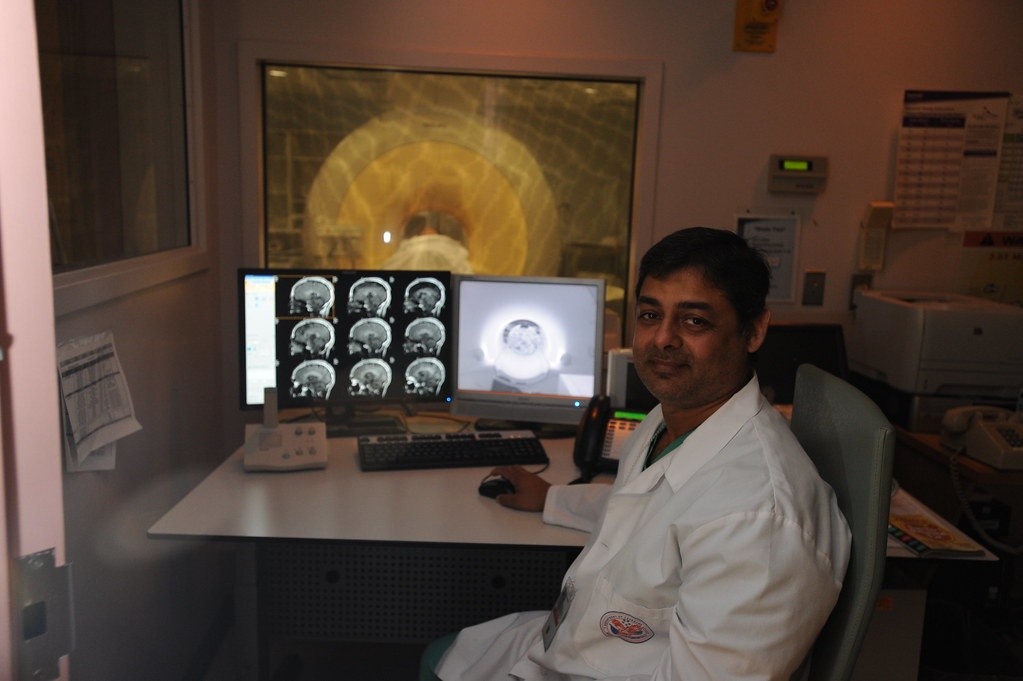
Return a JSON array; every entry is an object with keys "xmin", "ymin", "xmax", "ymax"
[{"xmin": 779, "ymin": 364, "xmax": 900, "ymax": 681}]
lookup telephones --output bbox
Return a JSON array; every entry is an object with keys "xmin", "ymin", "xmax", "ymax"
[{"xmin": 572, "ymin": 394, "xmax": 651, "ymax": 473}]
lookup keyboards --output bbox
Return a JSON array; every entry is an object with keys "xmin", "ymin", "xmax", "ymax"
[{"xmin": 358, "ymin": 430, "xmax": 549, "ymax": 471}]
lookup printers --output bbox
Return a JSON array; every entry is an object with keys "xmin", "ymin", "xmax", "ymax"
[{"xmin": 850, "ymin": 286, "xmax": 1023, "ymax": 400}]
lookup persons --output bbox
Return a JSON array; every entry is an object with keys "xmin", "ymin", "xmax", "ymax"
[{"xmin": 421, "ymin": 227, "xmax": 853, "ymax": 680}]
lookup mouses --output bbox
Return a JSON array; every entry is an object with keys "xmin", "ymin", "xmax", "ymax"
[{"xmin": 479, "ymin": 479, "xmax": 515, "ymax": 498}]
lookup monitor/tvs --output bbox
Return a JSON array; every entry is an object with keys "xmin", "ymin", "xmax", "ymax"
[
  {"xmin": 449, "ymin": 274, "xmax": 606, "ymax": 425},
  {"xmin": 238, "ymin": 268, "xmax": 452, "ymax": 435}
]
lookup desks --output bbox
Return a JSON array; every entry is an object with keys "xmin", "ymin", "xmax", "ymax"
[
  {"xmin": 895, "ymin": 426, "xmax": 1022, "ymax": 548},
  {"xmin": 147, "ymin": 409, "xmax": 1002, "ymax": 681}
]
